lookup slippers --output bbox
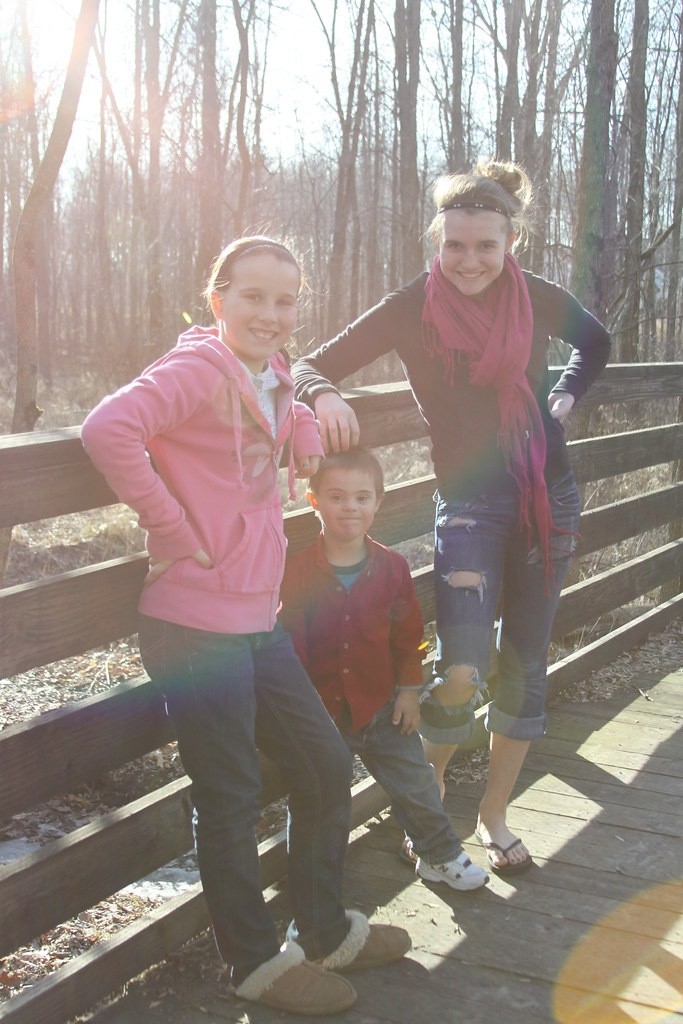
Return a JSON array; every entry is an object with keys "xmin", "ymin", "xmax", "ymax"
[
  {"xmin": 474, "ymin": 828, "xmax": 533, "ymax": 875},
  {"xmin": 399, "ymin": 828, "xmax": 421, "ymax": 865}
]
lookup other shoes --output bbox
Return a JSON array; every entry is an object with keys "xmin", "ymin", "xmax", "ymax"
[
  {"xmin": 229, "ymin": 941, "xmax": 358, "ymax": 1016},
  {"xmin": 308, "ymin": 910, "xmax": 412, "ymax": 972}
]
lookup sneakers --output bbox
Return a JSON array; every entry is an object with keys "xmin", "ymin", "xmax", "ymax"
[
  {"xmin": 415, "ymin": 850, "xmax": 487, "ymax": 890},
  {"xmin": 285, "ymin": 920, "xmax": 298, "ymax": 945}
]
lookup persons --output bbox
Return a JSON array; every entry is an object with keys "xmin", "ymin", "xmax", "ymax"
[
  {"xmin": 276, "ymin": 444, "xmax": 492, "ymax": 893},
  {"xmin": 287, "ymin": 156, "xmax": 613, "ymax": 879},
  {"xmin": 78, "ymin": 228, "xmax": 415, "ymax": 1015}
]
